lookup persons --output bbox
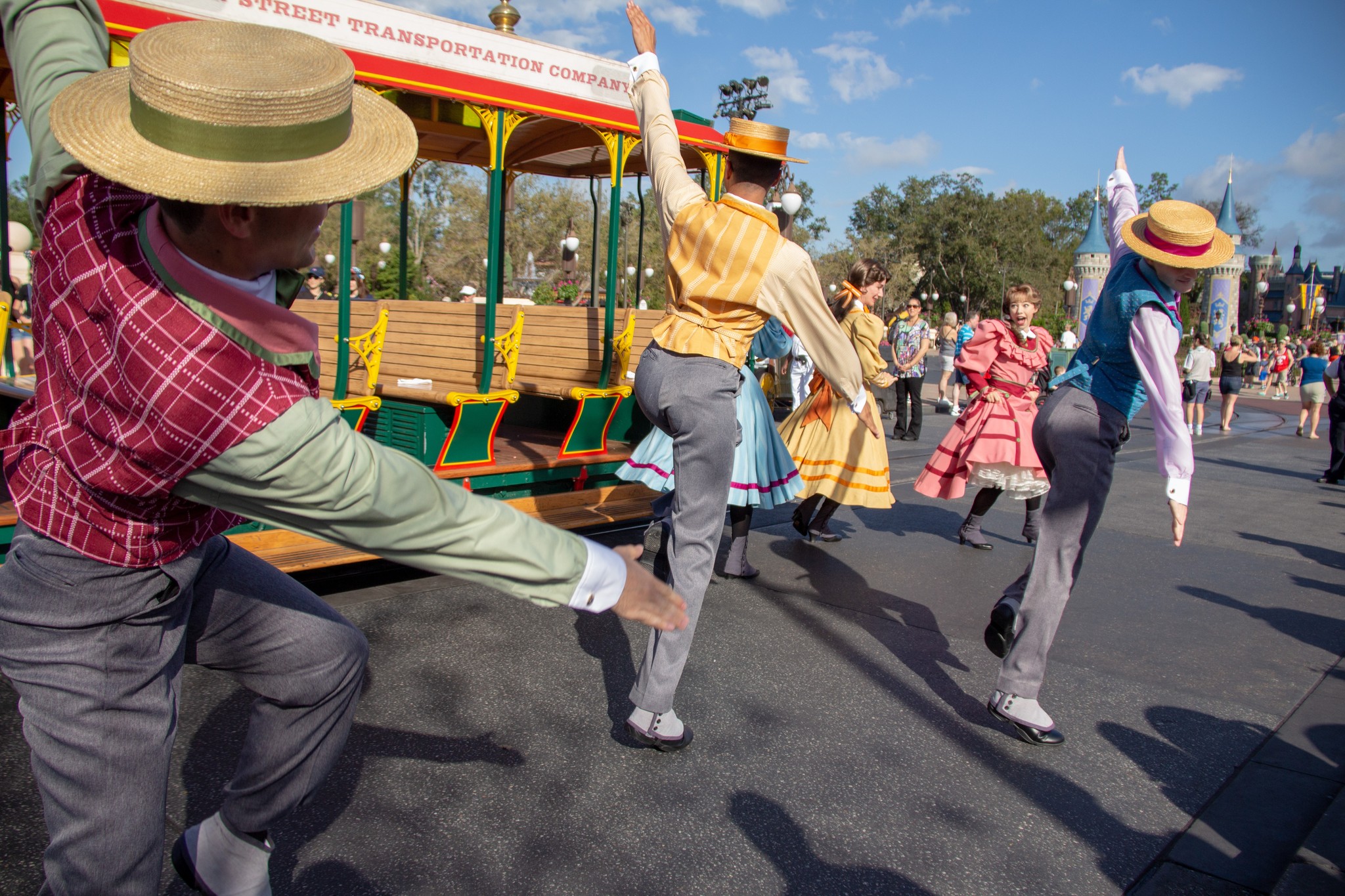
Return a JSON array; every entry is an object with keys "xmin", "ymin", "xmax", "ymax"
[
  {"xmin": 336, "ymin": 262, "xmax": 377, "ymax": 303},
  {"xmin": 976, "ymin": 145, "xmax": 1244, "ymax": 750},
  {"xmin": 13, "ymin": 262, "xmax": 38, "ymax": 377},
  {"xmin": 948, "ymin": 310, "xmax": 981, "ymax": 417},
  {"xmin": 458, "ymin": 281, "xmax": 478, "ymax": 305},
  {"xmin": 7, "ymin": 272, "xmax": 32, "ymax": 378},
  {"xmin": 935, "ymin": 311, "xmax": 957, "ymax": 406},
  {"xmin": 596, "ymin": 4, "xmax": 885, "ymax": 756},
  {"xmin": 781, "ymin": 256, "xmax": 898, "ymax": 545},
  {"xmin": 1053, "ymin": 323, "xmax": 1345, "ymax": 487},
  {"xmin": 934, "ymin": 324, "xmax": 940, "ymax": 348},
  {"xmin": 952, "ymin": 283, "xmax": 1054, "ymax": 552},
  {"xmin": 650, "ymin": 312, "xmax": 794, "ymax": 586},
  {"xmin": 782, "ymin": 326, "xmax": 816, "ymax": 413},
  {"xmin": 0, "ymin": 2, "xmax": 674, "ymax": 896},
  {"xmin": 889, "ymin": 297, "xmax": 931, "ymax": 442},
  {"xmin": 300, "ymin": 263, "xmax": 332, "ymax": 301}
]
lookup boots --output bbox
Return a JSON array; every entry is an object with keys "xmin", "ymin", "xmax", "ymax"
[
  {"xmin": 726, "ymin": 535, "xmax": 760, "ymax": 580},
  {"xmin": 807, "ymin": 502, "xmax": 842, "ymax": 544},
  {"xmin": 791, "ymin": 498, "xmax": 817, "ymax": 536},
  {"xmin": 957, "ymin": 512, "xmax": 994, "ymax": 550},
  {"xmin": 1022, "ymin": 507, "xmax": 1040, "ymax": 543}
]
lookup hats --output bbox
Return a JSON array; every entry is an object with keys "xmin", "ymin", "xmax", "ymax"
[
  {"xmin": 48, "ymin": 16, "xmax": 421, "ymax": 208},
  {"xmin": 702, "ymin": 117, "xmax": 809, "ymax": 165},
  {"xmin": 1121, "ymin": 200, "xmax": 1235, "ymax": 269}
]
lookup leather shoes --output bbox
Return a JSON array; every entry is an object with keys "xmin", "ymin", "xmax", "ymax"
[
  {"xmin": 903, "ymin": 436, "xmax": 915, "ymax": 441},
  {"xmin": 638, "ymin": 521, "xmax": 673, "ymax": 586},
  {"xmin": 624, "ymin": 721, "xmax": 694, "ymax": 753},
  {"xmin": 987, "ymin": 701, "xmax": 1065, "ymax": 746},
  {"xmin": 892, "ymin": 435, "xmax": 902, "ymax": 440},
  {"xmin": 984, "ymin": 603, "xmax": 1015, "ymax": 659},
  {"xmin": 1316, "ymin": 477, "xmax": 1338, "ymax": 484}
]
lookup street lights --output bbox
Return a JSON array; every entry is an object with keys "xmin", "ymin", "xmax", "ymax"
[
  {"xmin": 1062, "ymin": 265, "xmax": 1078, "ymax": 320},
  {"xmin": 1286, "ymin": 299, "xmax": 1296, "ymax": 335},
  {"xmin": 960, "ymin": 285, "xmax": 970, "ymax": 323},
  {"xmin": 370, "ymin": 235, "xmax": 392, "ymax": 297},
  {"xmin": 1335, "ymin": 314, "xmax": 1342, "ymax": 341},
  {"xmin": 922, "ymin": 271, "xmax": 940, "ymax": 319},
  {"xmin": 1313, "ymin": 287, "xmax": 1327, "ymax": 335},
  {"xmin": 560, "ymin": 215, "xmax": 579, "ymax": 306},
  {"xmin": 628, "ymin": 255, "xmax": 654, "ymax": 309},
  {"xmin": 1256, "ymin": 272, "xmax": 1270, "ymax": 321}
]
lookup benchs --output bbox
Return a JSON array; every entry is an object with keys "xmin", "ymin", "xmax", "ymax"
[
  {"xmin": 288, "ymin": 295, "xmax": 389, "ymax": 433},
  {"xmin": 604, "ymin": 309, "xmax": 667, "ymax": 444},
  {"xmin": 505, "ymin": 308, "xmax": 632, "ymax": 457},
  {"xmin": 384, "ymin": 298, "xmax": 524, "ymax": 470}
]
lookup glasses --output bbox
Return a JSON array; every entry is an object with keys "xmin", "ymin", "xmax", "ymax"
[{"xmin": 906, "ymin": 305, "xmax": 919, "ymax": 309}]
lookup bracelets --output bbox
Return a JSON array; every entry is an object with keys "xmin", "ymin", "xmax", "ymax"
[{"xmin": 909, "ymin": 362, "xmax": 913, "ymax": 369}]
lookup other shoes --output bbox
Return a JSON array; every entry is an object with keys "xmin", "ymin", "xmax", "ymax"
[
  {"xmin": 1296, "ymin": 426, "xmax": 1303, "ymax": 437},
  {"xmin": 1309, "ymin": 435, "xmax": 1319, "ymax": 439},
  {"xmin": 1220, "ymin": 425, "xmax": 1224, "ymax": 430},
  {"xmin": 1222, "ymin": 427, "xmax": 1231, "ymax": 431}
]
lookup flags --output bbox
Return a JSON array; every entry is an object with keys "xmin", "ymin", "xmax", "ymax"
[{"xmin": 1300, "ymin": 284, "xmax": 1323, "ymax": 330}]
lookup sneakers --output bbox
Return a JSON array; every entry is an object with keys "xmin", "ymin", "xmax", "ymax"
[
  {"xmin": 1188, "ymin": 428, "xmax": 1193, "ymax": 435},
  {"xmin": 1193, "ymin": 428, "xmax": 1202, "ymax": 435},
  {"xmin": 951, "ymin": 407, "xmax": 965, "ymax": 416},
  {"xmin": 939, "ymin": 399, "xmax": 954, "ymax": 406}
]
